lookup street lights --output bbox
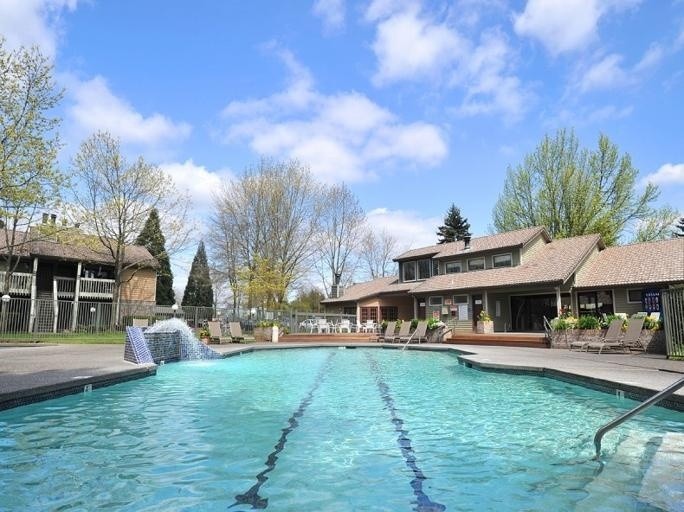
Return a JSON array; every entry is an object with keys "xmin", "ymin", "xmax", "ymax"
[
  {"xmin": 90, "ymin": 306, "xmax": 96, "ymax": 333},
  {"xmin": 172, "ymin": 302, "xmax": 179, "ymax": 317},
  {"xmin": 1, "ymin": 295, "xmax": 11, "ymax": 333},
  {"xmin": 251, "ymin": 307, "xmax": 258, "ymax": 325}
]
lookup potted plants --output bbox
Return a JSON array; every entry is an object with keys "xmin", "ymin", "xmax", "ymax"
[{"xmin": 475, "ymin": 310, "xmax": 495, "ymax": 335}]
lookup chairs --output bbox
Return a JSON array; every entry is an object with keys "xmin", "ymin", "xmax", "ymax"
[
  {"xmin": 207, "ymin": 319, "xmax": 257, "ymax": 345},
  {"xmin": 569, "ymin": 318, "xmax": 644, "ymax": 356},
  {"xmin": 377, "ymin": 319, "xmax": 429, "ymax": 344},
  {"xmin": 300, "ymin": 318, "xmax": 377, "ymax": 334}
]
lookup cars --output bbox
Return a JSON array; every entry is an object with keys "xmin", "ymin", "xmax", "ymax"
[{"xmin": 300, "ymin": 319, "xmax": 318, "ymax": 329}]
